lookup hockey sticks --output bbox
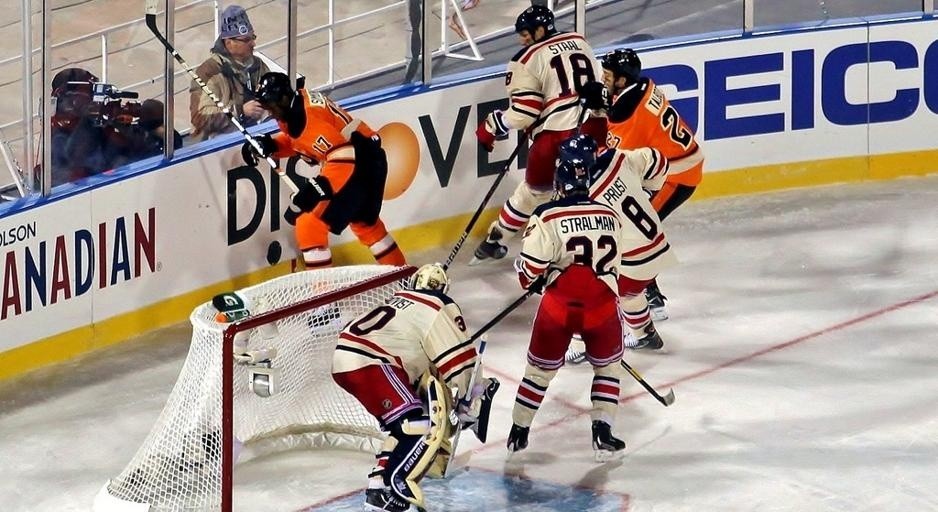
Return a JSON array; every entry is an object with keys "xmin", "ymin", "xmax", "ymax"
[
  {"xmin": 145, "ymin": 0, "xmax": 299, "ymax": 195},
  {"xmin": 621, "ymin": 359, "xmax": 675, "ymax": 406},
  {"xmin": 444, "ymin": 333, "xmax": 488, "ymax": 478}
]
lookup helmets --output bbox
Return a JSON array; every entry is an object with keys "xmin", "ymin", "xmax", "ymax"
[
  {"xmin": 600, "ymin": 48, "xmax": 642, "ymax": 83},
  {"xmin": 558, "ymin": 135, "xmax": 600, "ymax": 169},
  {"xmin": 407, "ymin": 262, "xmax": 452, "ymax": 296},
  {"xmin": 256, "ymin": 71, "xmax": 294, "ymax": 105},
  {"xmin": 552, "ymin": 154, "xmax": 591, "ymax": 193},
  {"xmin": 514, "ymin": 3, "xmax": 558, "ymax": 43}
]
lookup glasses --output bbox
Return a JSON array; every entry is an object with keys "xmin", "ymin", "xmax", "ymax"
[{"xmin": 231, "ymin": 35, "xmax": 258, "ymax": 43}]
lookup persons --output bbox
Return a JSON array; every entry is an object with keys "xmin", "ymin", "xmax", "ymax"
[
  {"xmin": 559, "ymin": 133, "xmax": 673, "ymax": 365},
  {"xmin": 190, "ymin": 6, "xmax": 273, "ymax": 145},
  {"xmin": 126, "ymin": 98, "xmax": 183, "ymax": 164},
  {"xmin": 506, "ymin": 152, "xmax": 626, "ymax": 451},
  {"xmin": 51, "ymin": 68, "xmax": 126, "ymax": 188},
  {"xmin": 474, "ymin": 5, "xmax": 608, "ymax": 260},
  {"xmin": 580, "ymin": 48, "xmax": 705, "ymax": 311},
  {"xmin": 331, "ymin": 263, "xmax": 483, "ymax": 512},
  {"xmin": 242, "ymin": 72, "xmax": 411, "ymax": 328}
]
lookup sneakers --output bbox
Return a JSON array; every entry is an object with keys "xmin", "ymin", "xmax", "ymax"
[
  {"xmin": 473, "ymin": 234, "xmax": 510, "ymax": 264},
  {"xmin": 590, "ymin": 418, "xmax": 627, "ymax": 452},
  {"xmin": 565, "ymin": 337, "xmax": 589, "ymax": 364},
  {"xmin": 644, "ymin": 281, "xmax": 665, "ymax": 311},
  {"xmin": 505, "ymin": 423, "xmax": 531, "ymax": 454},
  {"xmin": 364, "ymin": 486, "xmax": 415, "ymax": 512},
  {"xmin": 624, "ymin": 332, "xmax": 665, "ymax": 351}
]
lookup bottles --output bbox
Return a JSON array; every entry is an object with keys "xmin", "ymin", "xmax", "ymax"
[{"xmin": 215, "ymin": 308, "xmax": 252, "ymax": 325}]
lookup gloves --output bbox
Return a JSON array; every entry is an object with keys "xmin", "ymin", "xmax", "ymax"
[
  {"xmin": 289, "ymin": 175, "xmax": 335, "ymax": 216},
  {"xmin": 578, "ymin": 79, "xmax": 613, "ymax": 117},
  {"xmin": 513, "ymin": 256, "xmax": 545, "ymax": 298},
  {"xmin": 453, "ymin": 391, "xmax": 483, "ymax": 424},
  {"xmin": 241, "ymin": 131, "xmax": 277, "ymax": 168},
  {"xmin": 474, "ymin": 108, "xmax": 508, "ymax": 152}
]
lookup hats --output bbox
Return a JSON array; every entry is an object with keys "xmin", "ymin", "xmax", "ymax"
[{"xmin": 219, "ymin": 4, "xmax": 255, "ymax": 40}]
